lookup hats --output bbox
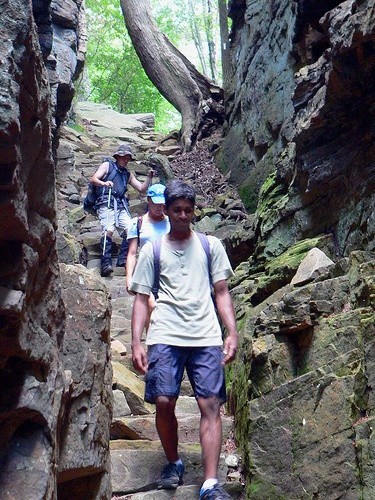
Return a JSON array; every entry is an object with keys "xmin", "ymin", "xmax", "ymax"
[
  {"xmin": 146, "ymin": 184, "xmax": 165, "ymax": 204},
  {"xmin": 112, "ymin": 145, "xmax": 136, "ymax": 162}
]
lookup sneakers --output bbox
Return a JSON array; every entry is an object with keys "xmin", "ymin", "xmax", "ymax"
[
  {"xmin": 157, "ymin": 463, "xmax": 184, "ymax": 489},
  {"xmin": 199, "ymin": 483, "xmax": 233, "ymax": 500}
]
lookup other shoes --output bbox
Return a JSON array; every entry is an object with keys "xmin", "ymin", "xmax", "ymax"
[
  {"xmin": 101, "ymin": 267, "xmax": 113, "ymax": 277},
  {"xmin": 115, "ymin": 259, "xmax": 126, "ymax": 267}
]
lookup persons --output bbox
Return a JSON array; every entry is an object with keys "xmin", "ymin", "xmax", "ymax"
[
  {"xmin": 90, "ymin": 144, "xmax": 156, "ymax": 277},
  {"xmin": 126, "ymin": 180, "xmax": 238, "ymax": 500},
  {"xmin": 126, "ymin": 184, "xmax": 171, "ymax": 335}
]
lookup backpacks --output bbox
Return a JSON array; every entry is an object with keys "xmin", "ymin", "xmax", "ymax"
[{"xmin": 82, "ymin": 159, "xmax": 127, "ymax": 216}]
